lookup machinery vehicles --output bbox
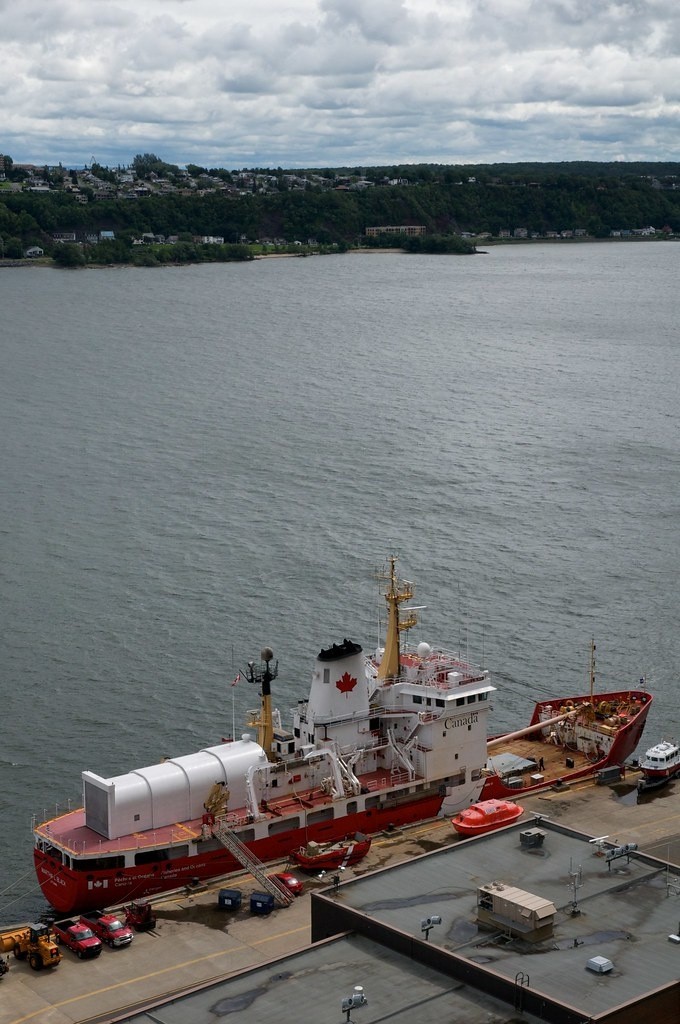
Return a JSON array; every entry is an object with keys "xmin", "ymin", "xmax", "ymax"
[
  {"xmin": 123, "ymin": 900, "xmax": 156, "ymax": 933},
  {"xmin": 1, "ymin": 922, "xmax": 63, "ymax": 972}
]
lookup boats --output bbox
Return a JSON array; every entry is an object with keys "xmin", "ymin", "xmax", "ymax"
[
  {"xmin": 636, "ymin": 741, "xmax": 680, "ymax": 795},
  {"xmin": 31, "ymin": 543, "xmax": 652, "ymax": 913},
  {"xmin": 450, "ymin": 796, "xmax": 525, "ymax": 837},
  {"xmin": 288, "ymin": 830, "xmax": 373, "ymax": 874}
]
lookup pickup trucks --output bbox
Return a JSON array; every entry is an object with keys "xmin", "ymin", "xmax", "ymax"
[
  {"xmin": 79, "ymin": 910, "xmax": 134, "ymax": 949},
  {"xmin": 52, "ymin": 918, "xmax": 102, "ymax": 961}
]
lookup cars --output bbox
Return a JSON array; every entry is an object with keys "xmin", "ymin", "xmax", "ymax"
[{"xmin": 265, "ymin": 873, "xmax": 303, "ymax": 895}]
